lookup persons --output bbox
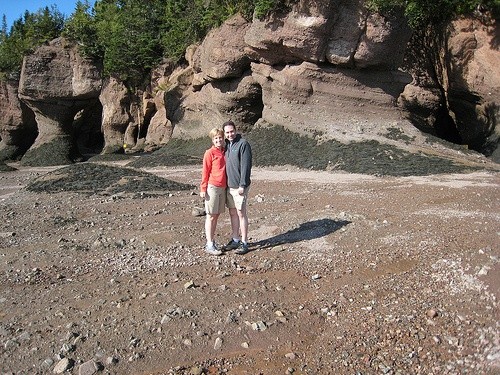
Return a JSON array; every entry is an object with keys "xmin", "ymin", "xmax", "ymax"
[
  {"xmin": 199, "ymin": 127, "xmax": 228, "ymax": 256},
  {"xmin": 223, "ymin": 121, "xmax": 253, "ymax": 254}
]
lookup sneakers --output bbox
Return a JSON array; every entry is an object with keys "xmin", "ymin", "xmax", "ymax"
[
  {"xmin": 205, "ymin": 244, "xmax": 222, "ymax": 255},
  {"xmin": 234, "ymin": 241, "xmax": 249, "ymax": 254},
  {"xmin": 223, "ymin": 238, "xmax": 241, "ymax": 250}
]
